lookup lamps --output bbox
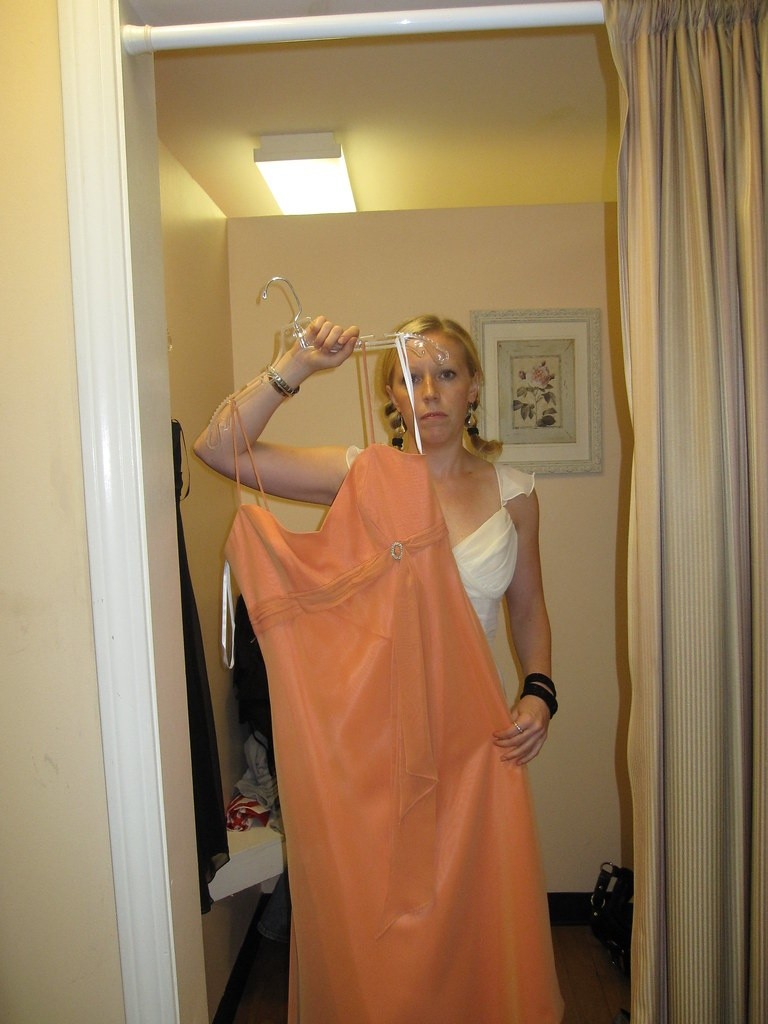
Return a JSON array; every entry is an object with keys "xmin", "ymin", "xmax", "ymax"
[{"xmin": 253, "ymin": 132, "xmax": 357, "ymax": 215}]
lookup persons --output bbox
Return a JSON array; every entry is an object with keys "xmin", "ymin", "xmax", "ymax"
[{"xmin": 193, "ymin": 316, "xmax": 554, "ymax": 1024}]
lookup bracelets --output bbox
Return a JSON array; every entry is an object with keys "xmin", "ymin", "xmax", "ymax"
[
  {"xmin": 268, "ymin": 368, "xmax": 300, "ymax": 398},
  {"xmin": 526, "ymin": 673, "xmax": 556, "ymax": 696},
  {"xmin": 521, "ymin": 683, "xmax": 558, "ymax": 719}
]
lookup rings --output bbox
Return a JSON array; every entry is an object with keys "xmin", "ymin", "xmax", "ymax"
[{"xmin": 514, "ymin": 722, "xmax": 523, "ymax": 733}]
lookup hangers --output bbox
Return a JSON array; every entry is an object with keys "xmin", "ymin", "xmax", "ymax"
[{"xmin": 207, "ymin": 277, "xmax": 449, "ymax": 452}]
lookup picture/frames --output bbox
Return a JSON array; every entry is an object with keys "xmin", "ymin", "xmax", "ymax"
[{"xmin": 469, "ymin": 307, "xmax": 604, "ymax": 475}]
